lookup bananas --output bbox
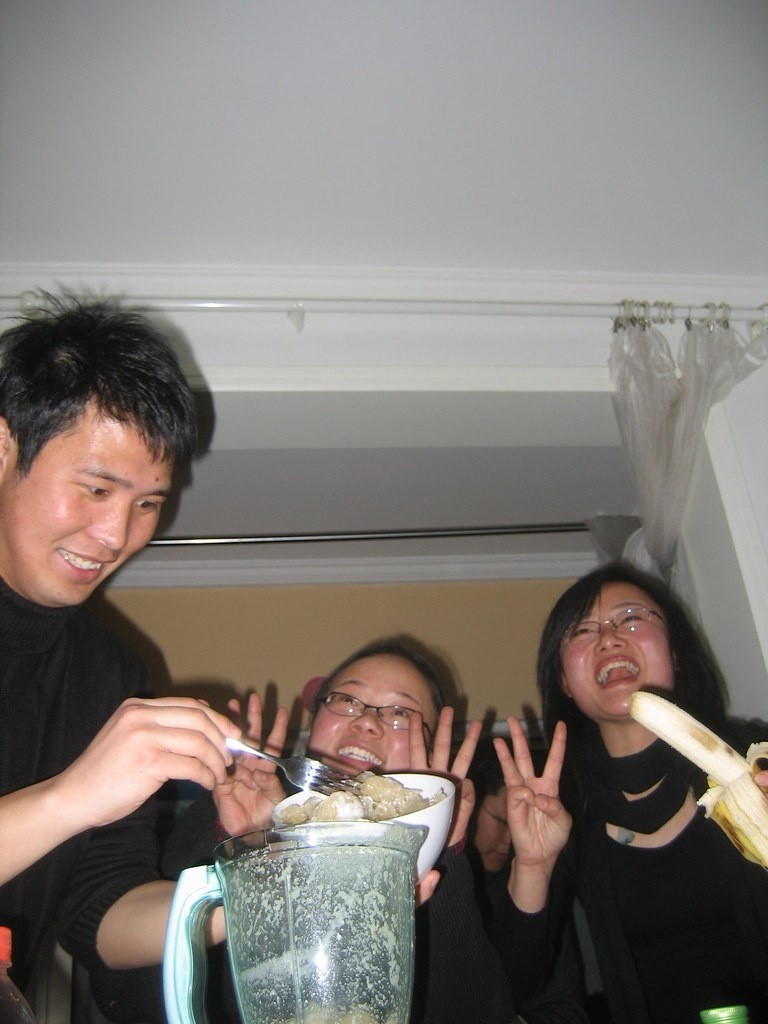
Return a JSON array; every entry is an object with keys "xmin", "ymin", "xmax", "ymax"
[{"xmin": 627, "ymin": 689, "xmax": 768, "ymax": 871}]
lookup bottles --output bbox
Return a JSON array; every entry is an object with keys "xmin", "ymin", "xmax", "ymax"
[{"xmin": 1, "ymin": 926, "xmax": 33, "ymax": 1023}]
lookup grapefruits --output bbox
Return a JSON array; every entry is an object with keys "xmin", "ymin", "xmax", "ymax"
[
  {"xmin": 279, "ymin": 999, "xmax": 381, "ymax": 1024},
  {"xmin": 278, "ymin": 770, "xmax": 447, "ymax": 822}
]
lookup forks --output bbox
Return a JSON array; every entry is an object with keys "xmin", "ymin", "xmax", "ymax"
[{"xmin": 222, "ymin": 734, "xmax": 362, "ymax": 799}]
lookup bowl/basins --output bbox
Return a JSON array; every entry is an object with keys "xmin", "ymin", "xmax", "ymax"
[{"xmin": 267, "ymin": 775, "xmax": 453, "ymax": 888}]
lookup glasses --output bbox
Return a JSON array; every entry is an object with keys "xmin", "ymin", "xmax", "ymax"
[
  {"xmin": 313, "ymin": 692, "xmax": 433, "ymax": 741},
  {"xmin": 559, "ymin": 609, "xmax": 673, "ymax": 641}
]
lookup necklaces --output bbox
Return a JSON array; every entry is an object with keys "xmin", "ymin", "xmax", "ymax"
[{"xmin": 615, "ymin": 826, "xmax": 635, "ymax": 845}]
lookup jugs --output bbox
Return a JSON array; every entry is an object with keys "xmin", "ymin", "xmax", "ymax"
[{"xmin": 163, "ymin": 824, "xmax": 429, "ymax": 1024}]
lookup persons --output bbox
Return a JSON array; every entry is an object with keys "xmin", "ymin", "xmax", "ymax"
[
  {"xmin": 0, "ymin": 284, "xmax": 443, "ymax": 991},
  {"xmin": 494, "ymin": 563, "xmax": 768, "ymax": 1024},
  {"xmin": 88, "ymin": 633, "xmax": 510, "ymax": 1024},
  {"xmin": 466, "ymin": 768, "xmax": 514, "ymax": 916}
]
